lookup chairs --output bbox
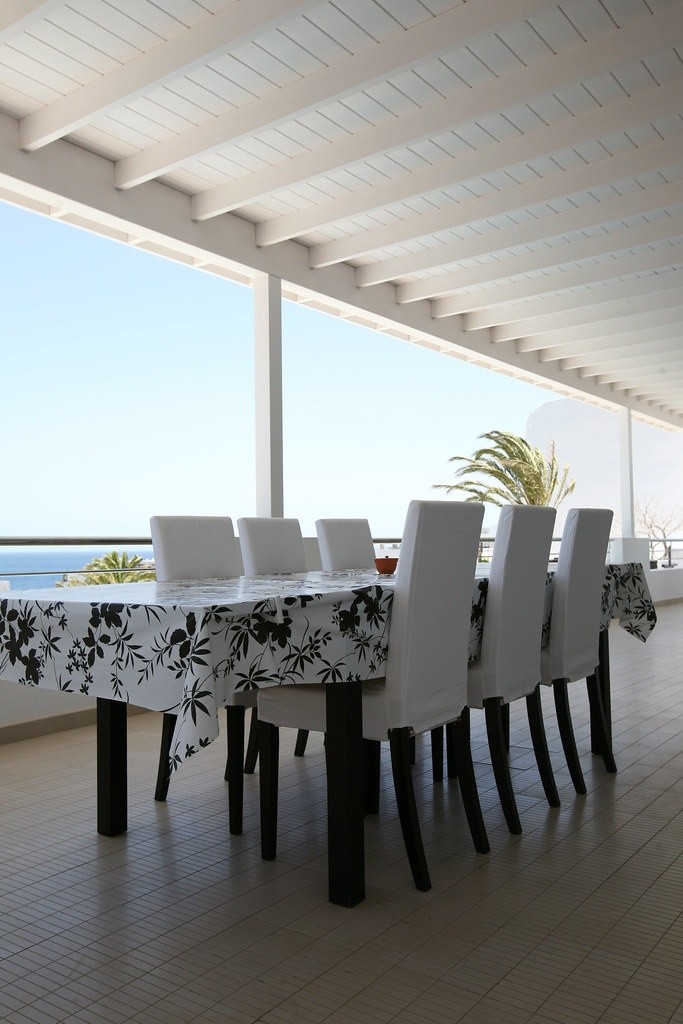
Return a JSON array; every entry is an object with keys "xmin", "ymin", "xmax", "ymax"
[
  {"xmin": 293, "ymin": 518, "xmax": 495, "ymax": 784},
  {"xmin": 431, "ymin": 507, "xmax": 618, "ymax": 797},
  {"xmin": 256, "ymin": 499, "xmax": 490, "ymax": 893},
  {"xmin": 221, "ymin": 516, "xmax": 415, "ymax": 792},
  {"xmin": 151, "ymin": 514, "xmax": 245, "ymax": 838},
  {"xmin": 365, "ymin": 504, "xmax": 562, "ymax": 835}
]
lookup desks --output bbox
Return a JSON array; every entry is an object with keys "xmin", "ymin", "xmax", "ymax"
[{"xmin": 5, "ymin": 559, "xmax": 648, "ymax": 837}]
